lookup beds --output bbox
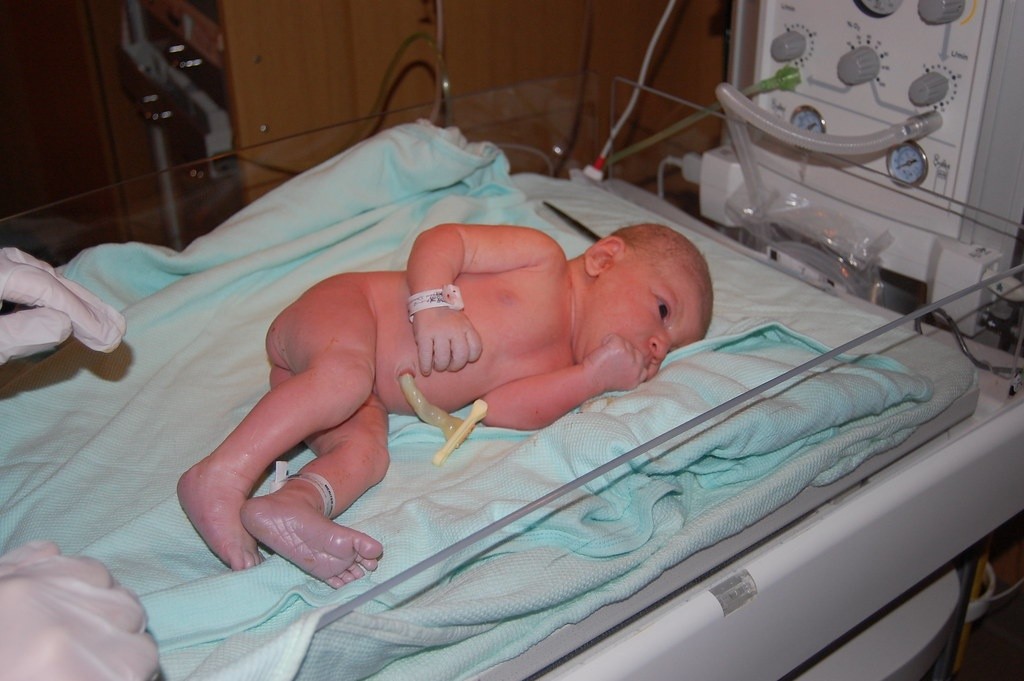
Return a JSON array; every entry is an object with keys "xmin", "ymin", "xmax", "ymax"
[{"xmin": 1, "ymin": 176, "xmax": 1024, "ymax": 678}]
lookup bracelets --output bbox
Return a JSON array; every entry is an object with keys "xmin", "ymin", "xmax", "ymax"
[{"xmin": 405, "ymin": 286, "xmax": 465, "ymax": 322}]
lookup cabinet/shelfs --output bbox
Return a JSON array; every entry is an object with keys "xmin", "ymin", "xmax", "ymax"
[{"xmin": 0, "ymin": 0, "xmax": 681, "ymax": 315}]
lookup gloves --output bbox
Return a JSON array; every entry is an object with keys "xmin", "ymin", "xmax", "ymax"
[
  {"xmin": 0, "ymin": 247, "xmax": 126, "ymax": 366},
  {"xmin": 0, "ymin": 541, "xmax": 160, "ymax": 681}
]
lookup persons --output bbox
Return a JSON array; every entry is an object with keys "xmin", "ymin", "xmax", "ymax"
[
  {"xmin": 0, "ymin": 245, "xmax": 160, "ymax": 681},
  {"xmin": 175, "ymin": 222, "xmax": 714, "ymax": 591}
]
